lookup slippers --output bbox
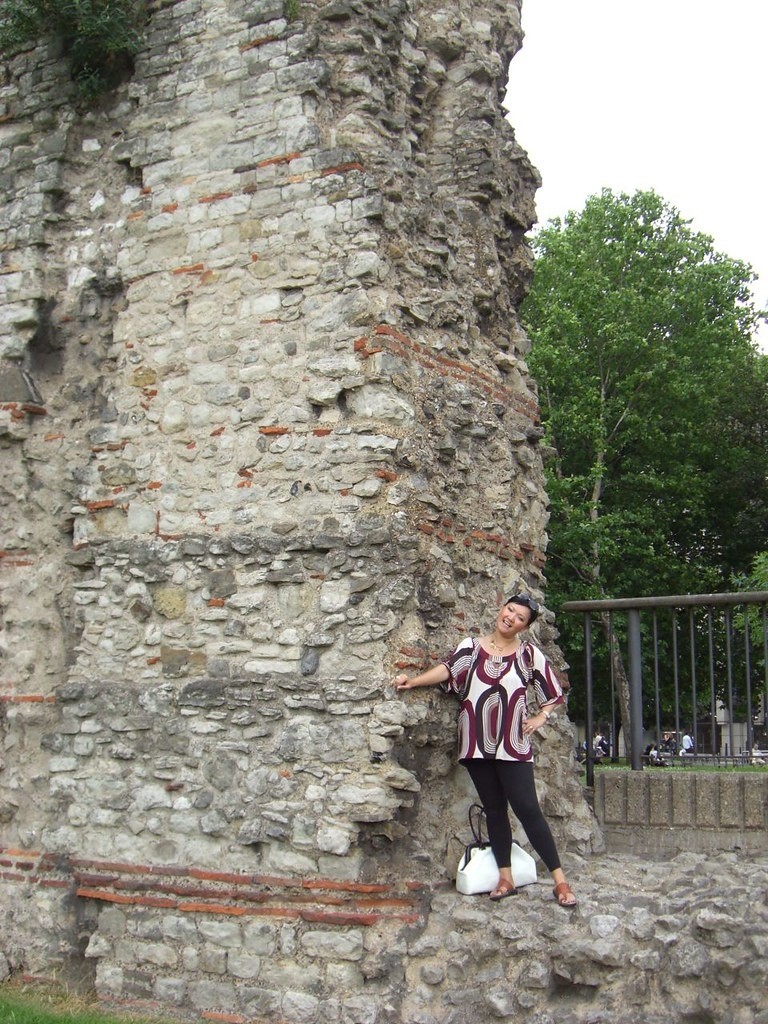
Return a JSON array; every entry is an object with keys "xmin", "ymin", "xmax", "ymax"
[
  {"xmin": 489, "ymin": 878, "xmax": 518, "ymax": 900},
  {"xmin": 553, "ymin": 883, "xmax": 577, "ymax": 907}
]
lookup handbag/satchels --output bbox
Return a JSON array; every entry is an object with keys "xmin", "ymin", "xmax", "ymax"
[{"xmin": 455, "ymin": 803, "xmax": 538, "ymax": 896}]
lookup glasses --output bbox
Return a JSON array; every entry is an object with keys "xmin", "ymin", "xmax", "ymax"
[{"xmin": 504, "ymin": 593, "xmax": 539, "ymax": 610}]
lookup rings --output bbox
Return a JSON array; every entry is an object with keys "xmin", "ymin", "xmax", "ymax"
[
  {"xmin": 530, "ymin": 726, "xmax": 532, "ymax": 729},
  {"xmin": 528, "ymin": 725, "xmax": 529, "ymax": 727},
  {"xmin": 396, "ymin": 676, "xmax": 400, "ymax": 679},
  {"xmin": 533, "ymin": 729, "xmax": 535, "ymax": 731}
]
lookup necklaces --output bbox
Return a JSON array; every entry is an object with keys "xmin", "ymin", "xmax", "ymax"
[{"xmin": 490, "ymin": 633, "xmax": 515, "ymax": 652}]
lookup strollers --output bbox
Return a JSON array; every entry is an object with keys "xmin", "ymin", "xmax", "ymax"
[{"xmin": 577, "ymin": 735, "xmax": 608, "ymax": 767}]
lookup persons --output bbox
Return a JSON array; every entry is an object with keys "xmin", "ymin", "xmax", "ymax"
[
  {"xmin": 578, "ymin": 732, "xmax": 608, "ymax": 765},
  {"xmin": 751, "ymin": 744, "xmax": 766, "ymax": 767},
  {"xmin": 392, "ymin": 594, "xmax": 578, "ymax": 907},
  {"xmin": 682, "ymin": 732, "xmax": 694, "ymax": 753},
  {"xmin": 644, "ymin": 733, "xmax": 677, "ymax": 766}
]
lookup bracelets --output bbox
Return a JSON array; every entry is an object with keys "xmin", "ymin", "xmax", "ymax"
[{"xmin": 541, "ymin": 710, "xmax": 551, "ymax": 719}]
